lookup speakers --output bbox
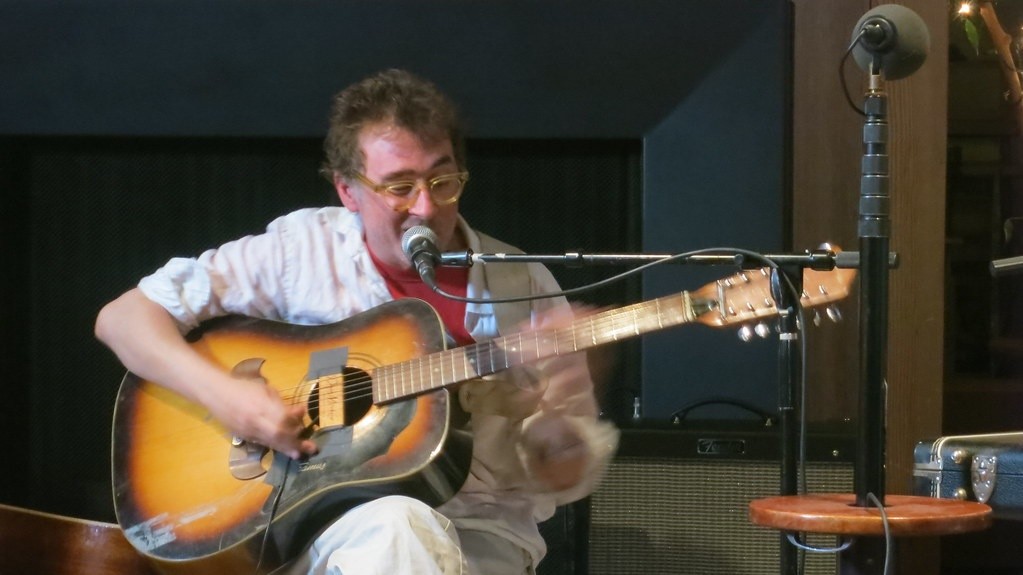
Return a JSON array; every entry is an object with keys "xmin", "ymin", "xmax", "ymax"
[{"xmin": 567, "ymin": 418, "xmax": 860, "ymax": 575}]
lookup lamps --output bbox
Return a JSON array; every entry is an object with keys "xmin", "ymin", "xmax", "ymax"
[{"xmin": 839, "ymin": 4, "xmax": 932, "ymax": 118}]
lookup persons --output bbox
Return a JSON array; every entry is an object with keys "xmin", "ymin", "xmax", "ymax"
[{"xmin": 93, "ymin": 67, "xmax": 620, "ymax": 575}]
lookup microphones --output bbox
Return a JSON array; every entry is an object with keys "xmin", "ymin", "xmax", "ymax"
[
  {"xmin": 852, "ymin": 4, "xmax": 930, "ymax": 82},
  {"xmin": 400, "ymin": 225, "xmax": 438, "ymax": 289}
]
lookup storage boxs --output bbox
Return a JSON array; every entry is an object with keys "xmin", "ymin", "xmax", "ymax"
[{"xmin": 913, "ymin": 438, "xmax": 1023, "ymax": 512}]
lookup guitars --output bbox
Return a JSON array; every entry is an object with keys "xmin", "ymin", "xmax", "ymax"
[{"xmin": 110, "ymin": 242, "xmax": 863, "ymax": 568}]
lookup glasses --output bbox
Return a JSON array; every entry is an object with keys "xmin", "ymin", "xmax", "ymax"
[{"xmin": 342, "ymin": 165, "xmax": 468, "ymax": 210}]
